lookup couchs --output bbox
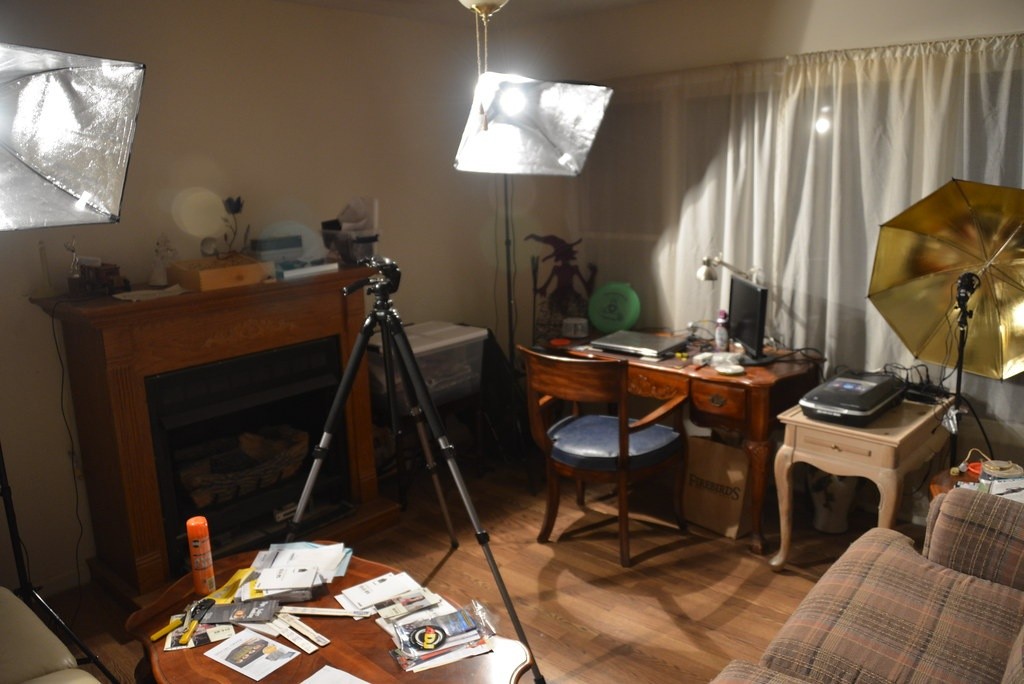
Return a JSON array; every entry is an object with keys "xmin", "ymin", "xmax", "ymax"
[
  {"xmin": 711, "ymin": 490, "xmax": 1024, "ymax": 684},
  {"xmin": 1, "ymin": 584, "xmax": 98, "ymax": 684}
]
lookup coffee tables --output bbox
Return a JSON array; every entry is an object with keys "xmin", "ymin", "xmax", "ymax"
[{"xmin": 125, "ymin": 538, "xmax": 533, "ymax": 684}]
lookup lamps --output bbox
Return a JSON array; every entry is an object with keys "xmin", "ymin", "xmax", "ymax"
[{"xmin": 694, "ymin": 256, "xmax": 752, "ymax": 289}]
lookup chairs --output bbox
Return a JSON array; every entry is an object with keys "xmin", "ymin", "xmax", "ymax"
[{"xmin": 516, "ymin": 345, "xmax": 688, "ymax": 567}]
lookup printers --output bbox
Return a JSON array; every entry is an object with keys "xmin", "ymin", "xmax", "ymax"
[{"xmin": 799, "ymin": 371, "xmax": 908, "ymax": 427}]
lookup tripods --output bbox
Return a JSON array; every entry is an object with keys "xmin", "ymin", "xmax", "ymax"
[{"xmin": 282, "ymin": 255, "xmax": 547, "ymax": 684}]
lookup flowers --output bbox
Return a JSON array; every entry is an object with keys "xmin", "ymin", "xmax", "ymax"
[{"xmin": 212, "ymin": 197, "xmax": 245, "ymax": 258}]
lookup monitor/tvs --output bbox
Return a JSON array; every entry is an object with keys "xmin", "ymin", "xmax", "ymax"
[{"xmin": 728, "ymin": 275, "xmax": 776, "ymax": 365}]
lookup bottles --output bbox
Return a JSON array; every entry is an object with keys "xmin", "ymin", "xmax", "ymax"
[
  {"xmin": 186, "ymin": 516, "xmax": 217, "ymax": 596},
  {"xmin": 715, "ymin": 310, "xmax": 729, "ymax": 351}
]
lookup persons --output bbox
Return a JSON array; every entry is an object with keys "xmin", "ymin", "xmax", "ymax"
[{"xmin": 397, "ymin": 596, "xmax": 425, "ymax": 607}]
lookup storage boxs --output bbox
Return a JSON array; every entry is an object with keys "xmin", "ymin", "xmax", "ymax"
[
  {"xmin": 317, "ymin": 226, "xmax": 379, "ymax": 265},
  {"xmin": 171, "ymin": 252, "xmax": 276, "ymax": 293},
  {"xmin": 678, "ymin": 438, "xmax": 758, "ymax": 540},
  {"xmin": 366, "ymin": 319, "xmax": 489, "ymax": 418}
]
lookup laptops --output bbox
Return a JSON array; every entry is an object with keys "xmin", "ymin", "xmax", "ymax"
[{"xmin": 591, "ymin": 330, "xmax": 689, "ymax": 357}]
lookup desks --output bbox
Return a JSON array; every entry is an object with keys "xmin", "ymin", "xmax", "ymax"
[{"xmin": 539, "ymin": 328, "xmax": 980, "ymax": 573}]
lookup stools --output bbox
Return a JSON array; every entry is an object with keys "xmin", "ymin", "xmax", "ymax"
[{"xmin": 370, "ymin": 391, "xmax": 492, "ymax": 511}]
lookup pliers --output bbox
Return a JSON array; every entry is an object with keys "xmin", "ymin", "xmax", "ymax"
[{"xmin": 150, "ymin": 599, "xmax": 215, "ymax": 645}]
